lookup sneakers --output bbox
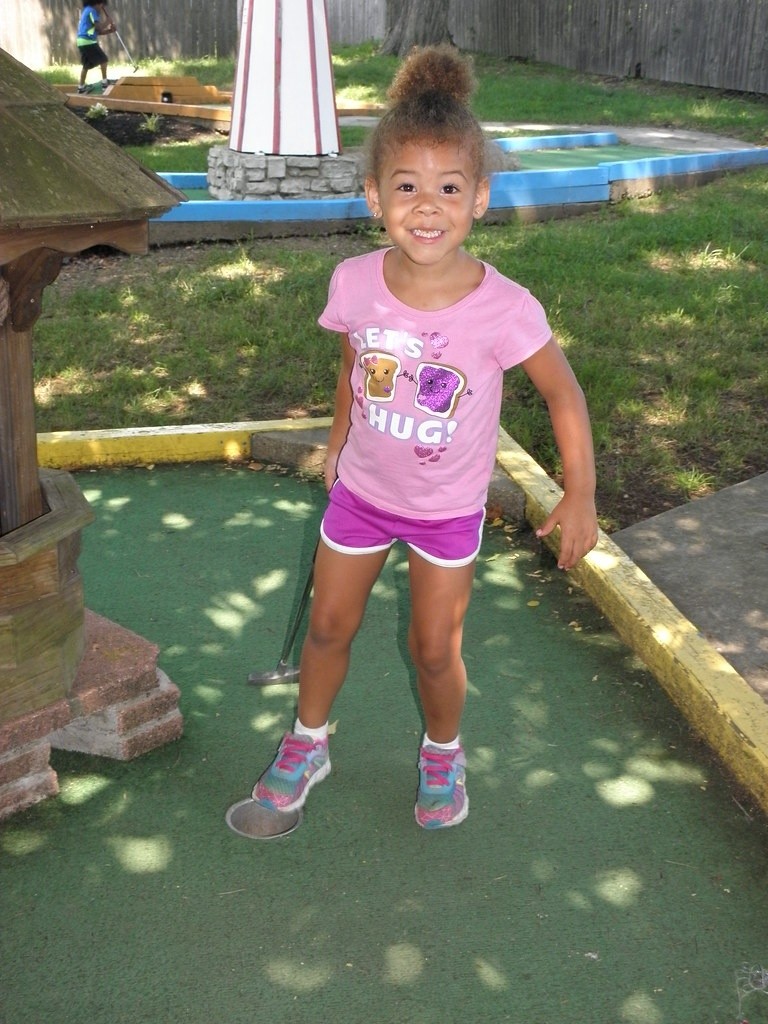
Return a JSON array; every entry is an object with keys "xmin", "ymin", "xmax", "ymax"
[
  {"xmin": 251, "ymin": 729, "xmax": 331, "ymax": 812},
  {"xmin": 414, "ymin": 743, "xmax": 469, "ymax": 830}
]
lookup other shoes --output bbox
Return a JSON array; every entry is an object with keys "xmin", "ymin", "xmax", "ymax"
[
  {"xmin": 79, "ymin": 84, "xmax": 95, "ymax": 94},
  {"xmin": 102, "ymin": 79, "xmax": 119, "ymax": 89}
]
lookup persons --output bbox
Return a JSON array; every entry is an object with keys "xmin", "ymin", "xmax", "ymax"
[
  {"xmin": 77, "ymin": 0, "xmax": 119, "ymax": 95},
  {"xmin": 252, "ymin": 45, "xmax": 598, "ymax": 828}
]
